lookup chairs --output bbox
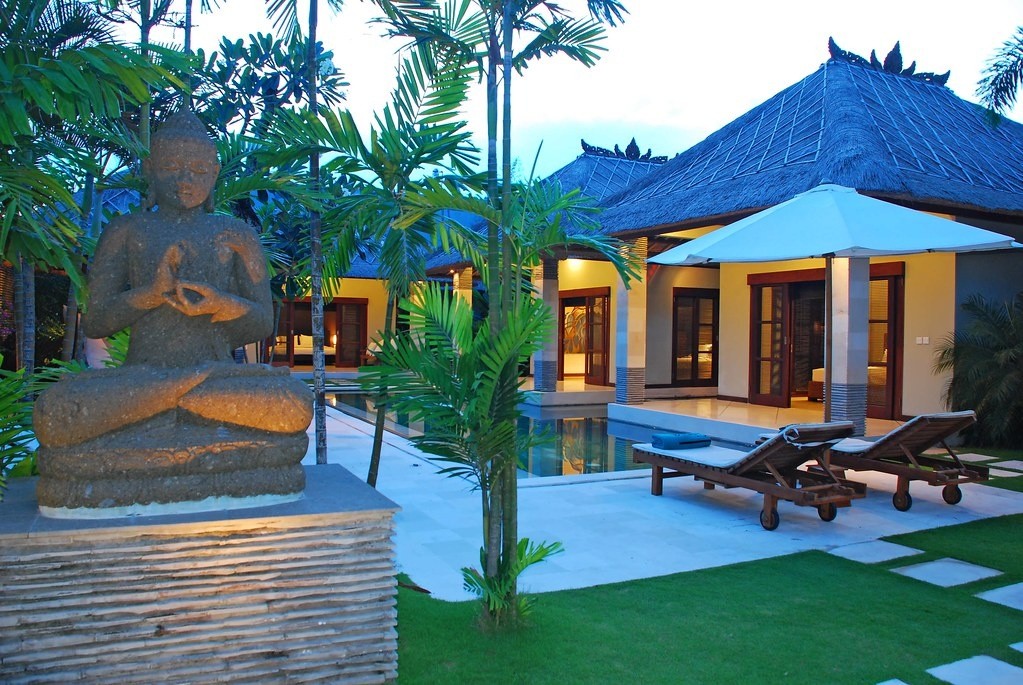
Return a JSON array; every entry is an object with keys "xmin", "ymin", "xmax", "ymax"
[
  {"xmin": 755, "ymin": 410, "xmax": 989, "ymax": 511},
  {"xmin": 633, "ymin": 420, "xmax": 868, "ymax": 531}
]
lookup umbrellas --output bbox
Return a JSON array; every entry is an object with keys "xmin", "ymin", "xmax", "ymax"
[{"xmin": 644, "ymin": 178, "xmax": 1023, "ymax": 465}]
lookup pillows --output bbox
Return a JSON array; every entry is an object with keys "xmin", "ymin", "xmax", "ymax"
[{"xmin": 276, "ymin": 335, "xmax": 313, "ymax": 348}]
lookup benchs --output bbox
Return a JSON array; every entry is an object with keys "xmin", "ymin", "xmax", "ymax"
[{"xmin": 361, "ymin": 335, "xmax": 398, "ymax": 367}]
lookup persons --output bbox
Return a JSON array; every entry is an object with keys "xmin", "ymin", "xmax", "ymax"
[{"xmin": 33, "ymin": 103, "xmax": 313, "ymax": 446}]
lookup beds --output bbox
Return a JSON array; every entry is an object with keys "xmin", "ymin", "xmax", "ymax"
[{"xmin": 269, "ymin": 345, "xmax": 336, "ymax": 364}]
